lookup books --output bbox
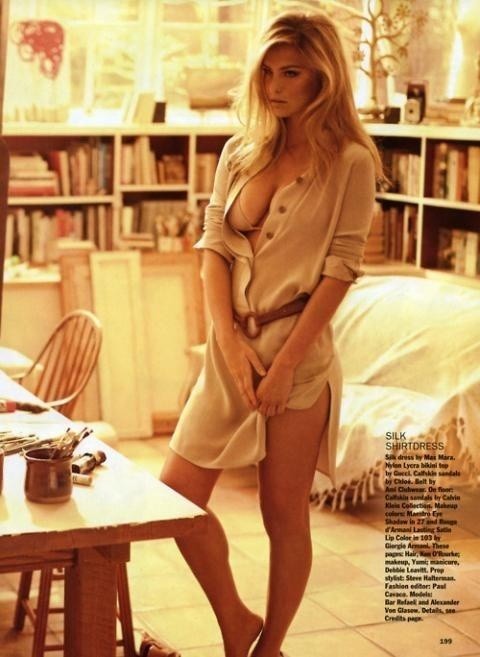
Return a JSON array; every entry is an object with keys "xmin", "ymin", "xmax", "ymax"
[
  {"xmin": 363, "ymin": 141, "xmax": 480, "ymax": 279},
  {"xmin": 4, "ymin": 135, "xmax": 221, "ymax": 268},
  {"xmin": 424, "ymin": 95, "xmax": 473, "ymax": 130}
]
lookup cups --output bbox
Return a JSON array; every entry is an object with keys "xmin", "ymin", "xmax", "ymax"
[{"xmin": 23, "ymin": 448, "xmax": 74, "ymax": 504}]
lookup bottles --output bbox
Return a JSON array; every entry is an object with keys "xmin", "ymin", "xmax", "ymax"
[{"xmin": 410, "ymin": 83, "xmax": 426, "ymax": 122}]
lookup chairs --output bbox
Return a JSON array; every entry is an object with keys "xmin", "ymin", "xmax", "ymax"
[{"xmin": 11, "ymin": 311, "xmax": 103, "ymax": 418}]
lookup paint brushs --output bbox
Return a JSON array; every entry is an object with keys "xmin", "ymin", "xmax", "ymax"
[
  {"xmin": 49, "ymin": 427, "xmax": 93, "ymax": 459},
  {"xmin": 0, "ymin": 434, "xmax": 62, "ymax": 455}
]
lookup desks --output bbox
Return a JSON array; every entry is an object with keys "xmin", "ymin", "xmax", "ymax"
[{"xmin": 1, "ymin": 370, "xmax": 209, "ymax": 657}]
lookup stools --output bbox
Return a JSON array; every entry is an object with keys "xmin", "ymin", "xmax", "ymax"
[{"xmin": 14, "ymin": 562, "xmax": 135, "ymax": 657}]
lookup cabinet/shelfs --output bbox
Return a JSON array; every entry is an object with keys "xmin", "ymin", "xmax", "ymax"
[{"xmin": 1, "ymin": 123, "xmax": 480, "ymax": 438}]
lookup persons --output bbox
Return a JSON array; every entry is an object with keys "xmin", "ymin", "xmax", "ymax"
[{"xmin": 159, "ymin": 8, "xmax": 384, "ymax": 657}]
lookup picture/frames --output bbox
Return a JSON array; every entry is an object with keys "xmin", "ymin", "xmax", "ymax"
[
  {"xmin": 141, "ymin": 253, "xmax": 206, "ymax": 434},
  {"xmin": 60, "ymin": 247, "xmax": 208, "ymax": 436}
]
